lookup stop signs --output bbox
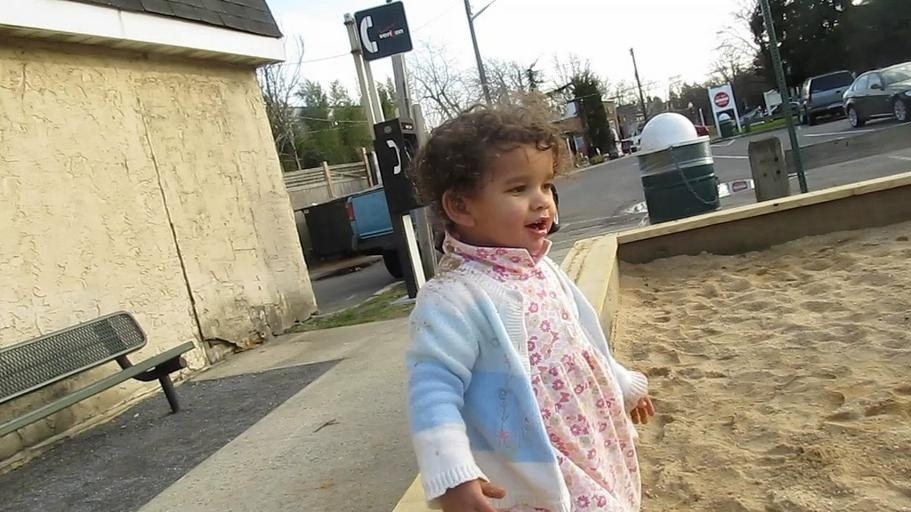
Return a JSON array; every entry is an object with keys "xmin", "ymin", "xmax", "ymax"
[{"xmin": 714, "ymin": 92, "xmax": 731, "ymax": 108}]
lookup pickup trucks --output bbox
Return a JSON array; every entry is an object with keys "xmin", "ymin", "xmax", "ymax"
[{"xmin": 301, "ymin": 176, "xmax": 562, "ymax": 280}]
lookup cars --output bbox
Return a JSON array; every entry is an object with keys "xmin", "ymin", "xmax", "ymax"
[
  {"xmin": 733, "ymin": 101, "xmax": 801, "ymax": 131},
  {"xmin": 841, "ymin": 61, "xmax": 911, "ymax": 128}
]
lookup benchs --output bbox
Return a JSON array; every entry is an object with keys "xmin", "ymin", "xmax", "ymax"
[{"xmin": 0, "ymin": 311, "xmax": 195, "ymax": 438}]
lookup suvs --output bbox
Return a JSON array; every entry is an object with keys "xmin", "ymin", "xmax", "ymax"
[{"xmin": 798, "ymin": 70, "xmax": 857, "ymax": 126}]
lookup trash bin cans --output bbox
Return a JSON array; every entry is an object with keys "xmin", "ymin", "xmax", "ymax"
[
  {"xmin": 633, "ymin": 111, "xmax": 722, "ymax": 227},
  {"xmin": 744, "ymin": 117, "xmax": 751, "ymax": 131},
  {"xmin": 588, "ymin": 145, "xmax": 604, "ymax": 165}
]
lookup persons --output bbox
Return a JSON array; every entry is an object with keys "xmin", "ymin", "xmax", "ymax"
[{"xmin": 394, "ymin": 85, "xmax": 657, "ymax": 512}]
